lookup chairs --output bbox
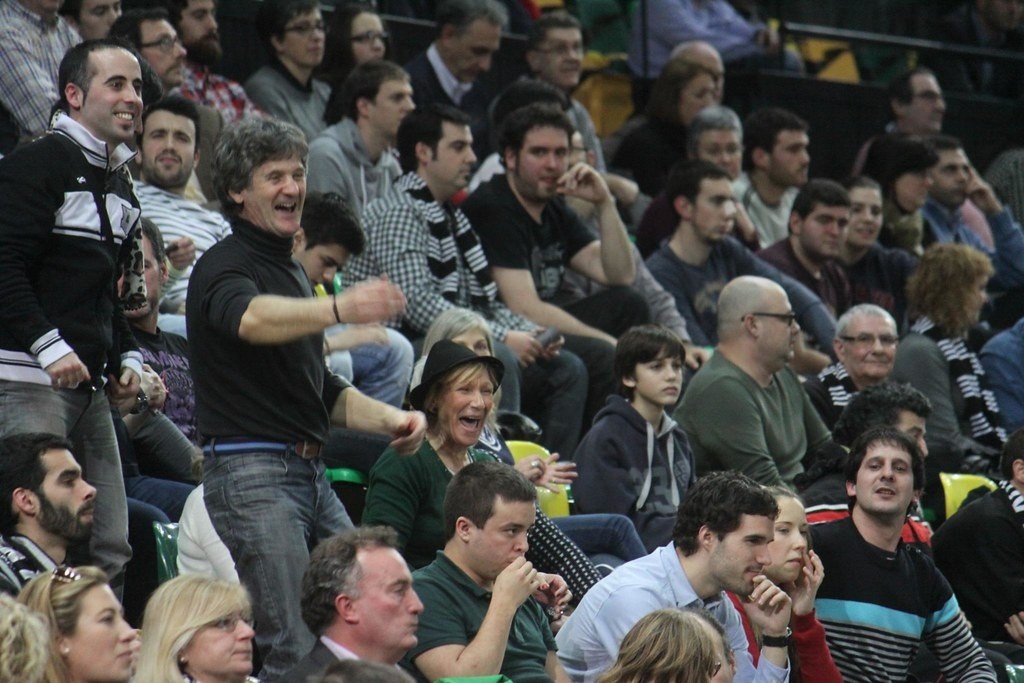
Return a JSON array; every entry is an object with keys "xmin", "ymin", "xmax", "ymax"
[{"xmin": 505, "ymin": 440, "xmax": 580, "ymax": 516}]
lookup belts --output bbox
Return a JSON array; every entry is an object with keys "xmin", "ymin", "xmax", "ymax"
[{"xmin": 202, "ymin": 440, "xmax": 326, "ymax": 459}]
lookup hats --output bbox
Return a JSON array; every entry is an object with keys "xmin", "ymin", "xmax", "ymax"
[{"xmin": 409, "ymin": 340, "xmax": 507, "ymax": 413}]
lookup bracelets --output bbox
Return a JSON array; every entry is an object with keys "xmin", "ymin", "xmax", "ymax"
[
  {"xmin": 761, "ymin": 628, "xmax": 792, "ymax": 648},
  {"xmin": 333, "ymin": 293, "xmax": 341, "ymax": 323}
]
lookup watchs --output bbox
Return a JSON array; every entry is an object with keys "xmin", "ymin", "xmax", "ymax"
[{"xmin": 130, "ymin": 386, "xmax": 149, "ymax": 414}]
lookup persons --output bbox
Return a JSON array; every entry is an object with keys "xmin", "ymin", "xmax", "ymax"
[
  {"xmin": 406, "ymin": 462, "xmax": 575, "ymax": 683},
  {"xmin": 0, "ymin": 0, "xmax": 1024, "ymax": 683},
  {"xmin": 132, "ymin": 572, "xmax": 260, "ymax": 683}
]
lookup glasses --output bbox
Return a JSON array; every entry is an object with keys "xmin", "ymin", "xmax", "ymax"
[
  {"xmin": 281, "ymin": 20, "xmax": 331, "ymax": 35},
  {"xmin": 208, "ymin": 613, "xmax": 255, "ymax": 633},
  {"xmin": 138, "ymin": 34, "xmax": 184, "ymax": 54},
  {"xmin": 535, "ymin": 43, "xmax": 584, "ymax": 57},
  {"xmin": 52, "ymin": 563, "xmax": 81, "ymax": 582},
  {"xmin": 741, "ymin": 312, "xmax": 796, "ymax": 327},
  {"xmin": 840, "ymin": 333, "xmax": 900, "ymax": 352},
  {"xmin": 700, "ymin": 143, "xmax": 745, "ymax": 156},
  {"xmin": 351, "ymin": 30, "xmax": 391, "ymax": 45}
]
